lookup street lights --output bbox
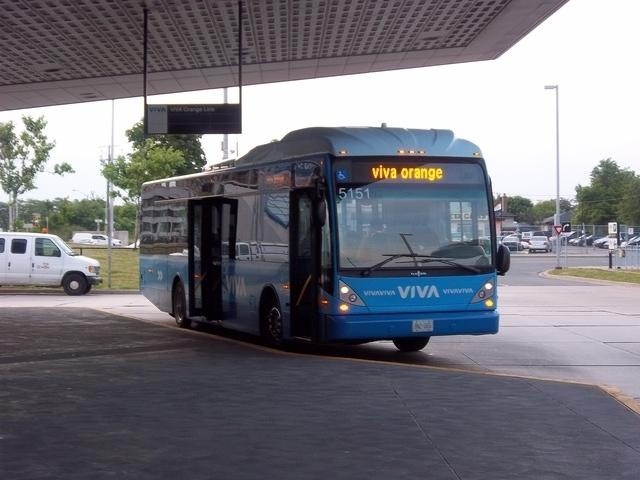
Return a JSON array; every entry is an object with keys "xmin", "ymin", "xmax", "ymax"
[
  {"xmin": 95, "ymin": 220, "xmax": 102, "ymax": 231},
  {"xmin": 544, "ymin": 86, "xmax": 562, "ymax": 269}
]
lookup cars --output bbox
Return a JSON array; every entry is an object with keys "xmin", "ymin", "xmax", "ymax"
[
  {"xmin": 70, "ymin": 233, "xmax": 121, "ymax": 246},
  {"xmin": 480, "ymin": 231, "xmax": 640, "ymax": 254}
]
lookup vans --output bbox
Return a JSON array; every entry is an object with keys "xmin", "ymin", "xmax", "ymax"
[
  {"xmin": 220, "ymin": 241, "xmax": 291, "ymax": 266},
  {"xmin": 0, "ymin": 232, "xmax": 102, "ymax": 295}
]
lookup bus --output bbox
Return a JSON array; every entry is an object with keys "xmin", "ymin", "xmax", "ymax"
[{"xmin": 139, "ymin": 123, "xmax": 510, "ymax": 354}]
